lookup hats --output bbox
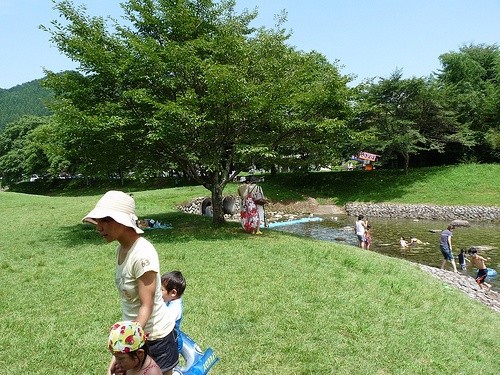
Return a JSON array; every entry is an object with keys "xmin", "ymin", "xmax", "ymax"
[
  {"xmin": 239, "ymin": 177, "xmax": 246, "ymax": 182},
  {"xmin": 108, "ymin": 321, "xmax": 150, "ymax": 353},
  {"xmin": 82, "ymin": 190, "xmax": 144, "ymax": 234}
]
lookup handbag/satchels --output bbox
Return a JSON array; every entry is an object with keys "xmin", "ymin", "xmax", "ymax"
[{"xmin": 254, "ymin": 186, "xmax": 266, "ymax": 205}]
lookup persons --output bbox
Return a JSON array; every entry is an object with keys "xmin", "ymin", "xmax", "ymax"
[
  {"xmin": 468, "ymin": 247, "xmax": 492, "ymax": 291},
  {"xmin": 410, "ymin": 235, "xmax": 420, "ymax": 243},
  {"xmin": 456, "ymin": 249, "xmax": 470, "ymax": 270},
  {"xmin": 439, "ymin": 226, "xmax": 457, "ymax": 273},
  {"xmin": 161, "ymin": 271, "xmax": 185, "ymax": 351},
  {"xmin": 82, "ymin": 190, "xmax": 179, "ymax": 375},
  {"xmin": 355, "ymin": 214, "xmax": 368, "ymax": 248},
  {"xmin": 365, "ymin": 224, "xmax": 372, "ymax": 249},
  {"xmin": 238, "ymin": 179, "xmax": 267, "ymax": 235},
  {"xmin": 399, "ymin": 236, "xmax": 408, "ymax": 248},
  {"xmin": 107, "ymin": 320, "xmax": 163, "ymax": 375}
]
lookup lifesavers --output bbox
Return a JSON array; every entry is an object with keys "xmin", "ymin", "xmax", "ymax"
[
  {"xmin": 178, "ymin": 330, "xmax": 216, "ymax": 375},
  {"xmin": 473, "ymin": 267, "xmax": 497, "ymax": 276}
]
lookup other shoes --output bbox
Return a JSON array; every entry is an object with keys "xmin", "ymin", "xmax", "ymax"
[
  {"xmin": 256, "ymin": 231, "xmax": 263, "ymax": 235},
  {"xmin": 252, "ymin": 231, "xmax": 255, "ymax": 234}
]
